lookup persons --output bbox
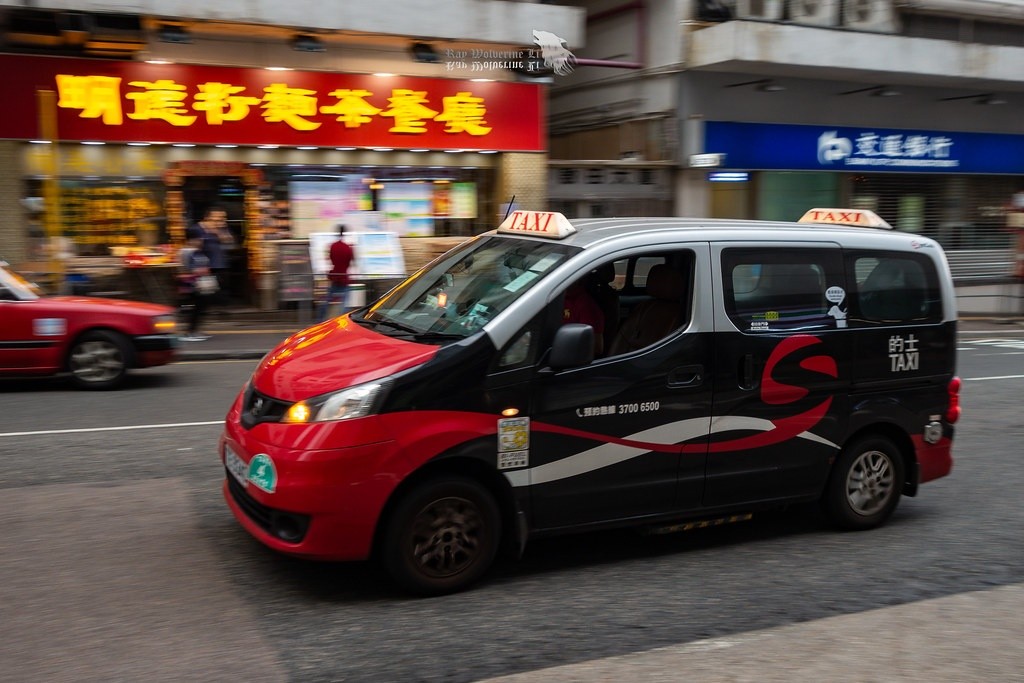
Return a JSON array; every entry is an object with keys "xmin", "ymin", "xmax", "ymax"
[
  {"xmin": 179, "ymin": 225, "xmax": 215, "ymax": 344},
  {"xmin": 193, "ymin": 205, "xmax": 241, "ymax": 319},
  {"xmin": 556, "ymin": 278, "xmax": 604, "ymax": 358},
  {"xmin": 316, "ymin": 222, "xmax": 354, "ymax": 322}
]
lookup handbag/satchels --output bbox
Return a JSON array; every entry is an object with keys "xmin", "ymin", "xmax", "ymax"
[{"xmin": 196, "ymin": 274, "xmax": 218, "ymax": 295}]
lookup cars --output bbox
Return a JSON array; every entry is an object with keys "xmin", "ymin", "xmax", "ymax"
[
  {"xmin": 217, "ymin": 206, "xmax": 964, "ymax": 596},
  {"xmin": 0, "ymin": 256, "xmax": 183, "ymax": 389}
]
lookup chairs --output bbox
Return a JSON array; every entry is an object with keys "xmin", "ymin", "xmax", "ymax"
[
  {"xmin": 546, "ymin": 262, "xmax": 618, "ymax": 361},
  {"xmin": 859, "ymin": 258, "xmax": 924, "ymax": 322},
  {"xmin": 755, "ymin": 262, "xmax": 821, "ymax": 312},
  {"xmin": 608, "ymin": 263, "xmax": 691, "ymax": 355}
]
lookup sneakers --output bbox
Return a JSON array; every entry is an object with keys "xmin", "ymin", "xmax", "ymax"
[{"xmin": 178, "ymin": 333, "xmax": 213, "ymax": 341}]
